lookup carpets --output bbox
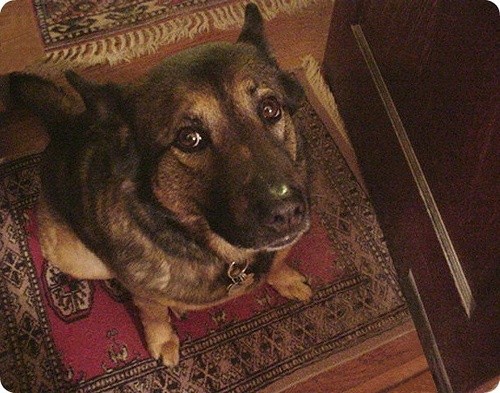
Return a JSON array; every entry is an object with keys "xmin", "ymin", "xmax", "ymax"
[
  {"xmin": 33, "ymin": 0, "xmax": 313, "ymax": 78},
  {"xmin": 0, "ymin": 54, "xmax": 418, "ymax": 393}
]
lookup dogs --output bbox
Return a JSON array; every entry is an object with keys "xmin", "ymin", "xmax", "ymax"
[{"xmin": 0, "ymin": 4, "xmax": 313, "ymax": 368}]
